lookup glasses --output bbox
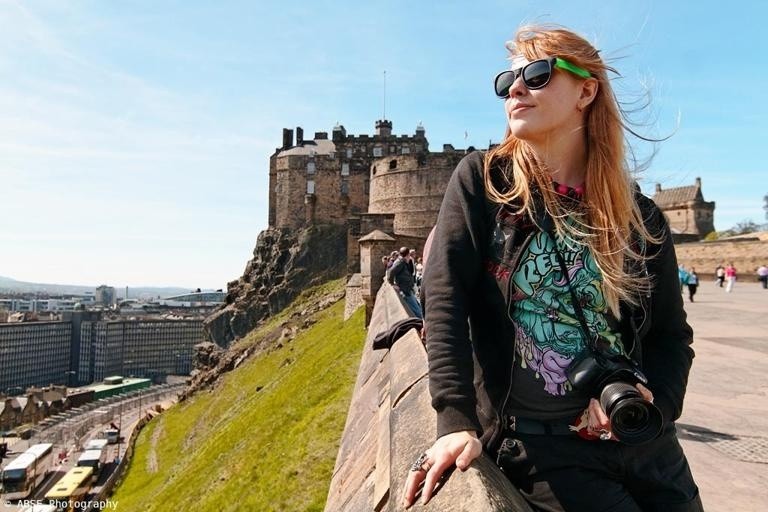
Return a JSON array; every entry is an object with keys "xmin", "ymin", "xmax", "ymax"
[{"xmin": 493, "ymin": 54, "xmax": 593, "ymax": 99}]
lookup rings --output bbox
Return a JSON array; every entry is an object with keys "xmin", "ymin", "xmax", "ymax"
[
  {"xmin": 598, "ymin": 426, "xmax": 615, "ymax": 440},
  {"xmin": 410, "ymin": 452, "xmax": 431, "ymax": 473}
]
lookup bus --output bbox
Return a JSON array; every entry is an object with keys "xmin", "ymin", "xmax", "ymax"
[{"xmin": 1, "ymin": 428, "xmax": 120, "ymax": 512}]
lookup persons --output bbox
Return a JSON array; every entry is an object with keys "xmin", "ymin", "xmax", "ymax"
[
  {"xmin": 382, "ymin": 247, "xmax": 422, "ymax": 318},
  {"xmin": 717, "ymin": 265, "xmax": 724, "ymax": 288},
  {"xmin": 725, "ymin": 262, "xmax": 737, "ymax": 292},
  {"xmin": 714, "ymin": 264, "xmax": 725, "ymax": 285},
  {"xmin": 757, "ymin": 264, "xmax": 767, "ymax": 288},
  {"xmin": 687, "ymin": 266, "xmax": 699, "ymax": 302},
  {"xmin": 397, "ymin": 19, "xmax": 710, "ymax": 511},
  {"xmin": 677, "ymin": 264, "xmax": 689, "ymax": 294}
]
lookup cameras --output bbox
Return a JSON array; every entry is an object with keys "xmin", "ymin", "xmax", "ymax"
[{"xmin": 565, "ymin": 335, "xmax": 663, "ymax": 446}]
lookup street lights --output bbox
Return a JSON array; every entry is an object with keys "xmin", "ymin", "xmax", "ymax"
[
  {"xmin": 136, "ymin": 388, "xmax": 142, "ymax": 421},
  {"xmin": 64, "ymin": 370, "xmax": 76, "ymax": 386},
  {"xmin": 175, "ymin": 353, "xmax": 180, "ymax": 379},
  {"xmin": 3, "ymin": 386, "xmax": 23, "ymax": 434},
  {"xmin": 124, "ymin": 359, "xmax": 132, "ymax": 382}
]
